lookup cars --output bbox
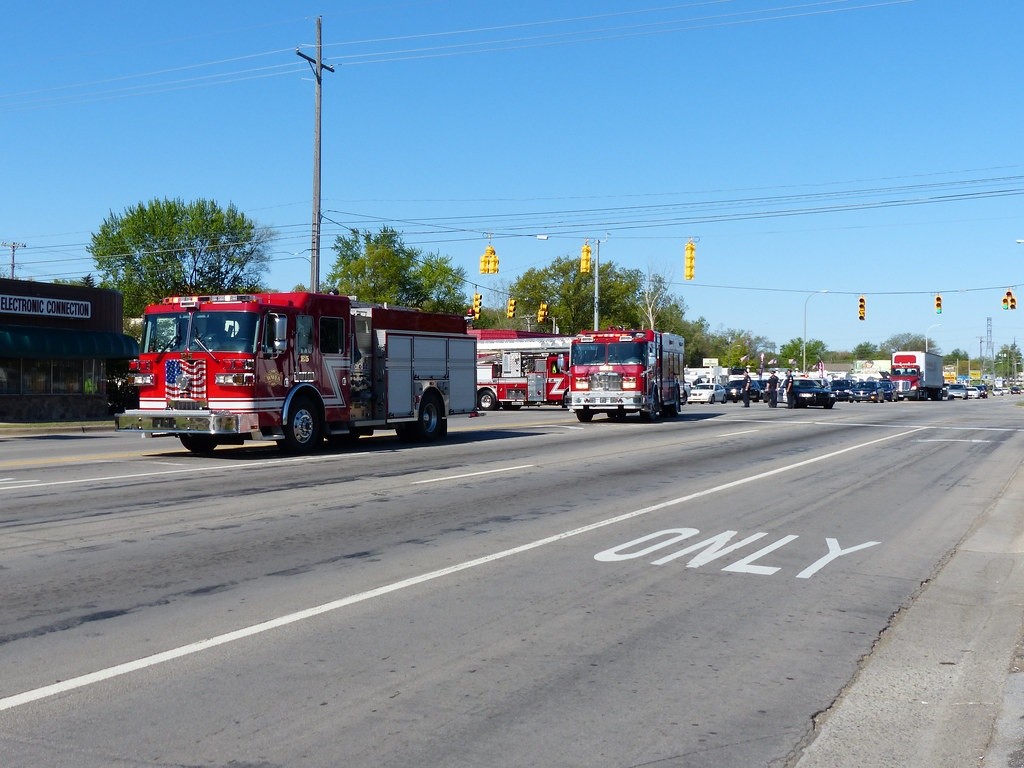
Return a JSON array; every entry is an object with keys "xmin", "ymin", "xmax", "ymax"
[
  {"xmin": 992, "ymin": 389, "xmax": 1003, "ymax": 396},
  {"xmin": 1002, "ymin": 388, "xmax": 1010, "ymax": 394},
  {"xmin": 687, "ymin": 383, "xmax": 727, "ymax": 404},
  {"xmin": 757, "ymin": 380, "xmax": 781, "ymax": 400},
  {"xmin": 847, "ymin": 381, "xmax": 885, "ymax": 403},
  {"xmin": 974, "ymin": 386, "xmax": 988, "ymax": 398},
  {"xmin": 815, "ymin": 378, "xmax": 856, "ymax": 401},
  {"xmin": 684, "ymin": 384, "xmax": 691, "ymax": 396},
  {"xmin": 724, "ymin": 380, "xmax": 761, "ymax": 402},
  {"xmin": 1010, "ymin": 387, "xmax": 1024, "ymax": 395},
  {"xmin": 943, "ymin": 383, "xmax": 950, "ymax": 397},
  {"xmin": 947, "ymin": 385, "xmax": 968, "ymax": 399},
  {"xmin": 766, "ymin": 374, "xmax": 836, "ymax": 408},
  {"xmin": 880, "ymin": 381, "xmax": 898, "ymax": 402},
  {"xmin": 967, "ymin": 387, "xmax": 981, "ymax": 399}
]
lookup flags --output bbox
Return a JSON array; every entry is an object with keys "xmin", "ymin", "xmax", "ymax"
[
  {"xmin": 788, "ymin": 359, "xmax": 796, "ymax": 365},
  {"xmin": 740, "ymin": 354, "xmax": 750, "ymax": 362},
  {"xmin": 767, "ymin": 358, "xmax": 777, "ymax": 365}
]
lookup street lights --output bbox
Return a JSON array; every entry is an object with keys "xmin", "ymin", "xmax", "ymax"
[
  {"xmin": 803, "ymin": 289, "xmax": 829, "ymax": 373},
  {"xmin": 925, "ymin": 323, "xmax": 941, "ymax": 353}
]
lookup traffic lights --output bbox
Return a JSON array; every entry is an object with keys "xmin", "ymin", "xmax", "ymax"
[
  {"xmin": 858, "ymin": 297, "xmax": 866, "ymax": 321},
  {"xmin": 935, "ymin": 296, "xmax": 942, "ymax": 315},
  {"xmin": 538, "ymin": 305, "xmax": 549, "ymax": 324},
  {"xmin": 507, "ymin": 298, "xmax": 516, "ymax": 319},
  {"xmin": 474, "ymin": 294, "xmax": 482, "ymax": 320},
  {"xmin": 1003, "ymin": 290, "xmax": 1017, "ymax": 310}
]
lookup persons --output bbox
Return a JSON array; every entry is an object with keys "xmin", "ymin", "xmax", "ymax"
[
  {"xmin": 782, "ymin": 369, "xmax": 794, "ymax": 409},
  {"xmin": 764, "ymin": 370, "xmax": 780, "ymax": 408},
  {"xmin": 741, "ymin": 370, "xmax": 751, "ymax": 407}
]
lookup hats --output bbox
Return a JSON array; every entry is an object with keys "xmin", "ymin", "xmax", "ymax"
[
  {"xmin": 744, "ymin": 370, "xmax": 748, "ymax": 373},
  {"xmin": 771, "ymin": 371, "xmax": 775, "ymax": 374},
  {"xmin": 787, "ymin": 370, "xmax": 793, "ymax": 372}
]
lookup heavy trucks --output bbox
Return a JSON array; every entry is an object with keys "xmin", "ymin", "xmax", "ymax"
[{"xmin": 889, "ymin": 351, "xmax": 944, "ymax": 401}]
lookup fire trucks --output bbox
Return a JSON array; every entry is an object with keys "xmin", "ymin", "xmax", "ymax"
[
  {"xmin": 557, "ymin": 329, "xmax": 686, "ymax": 422},
  {"xmin": 475, "ymin": 337, "xmax": 576, "ymax": 410},
  {"xmin": 114, "ymin": 292, "xmax": 486, "ymax": 454}
]
